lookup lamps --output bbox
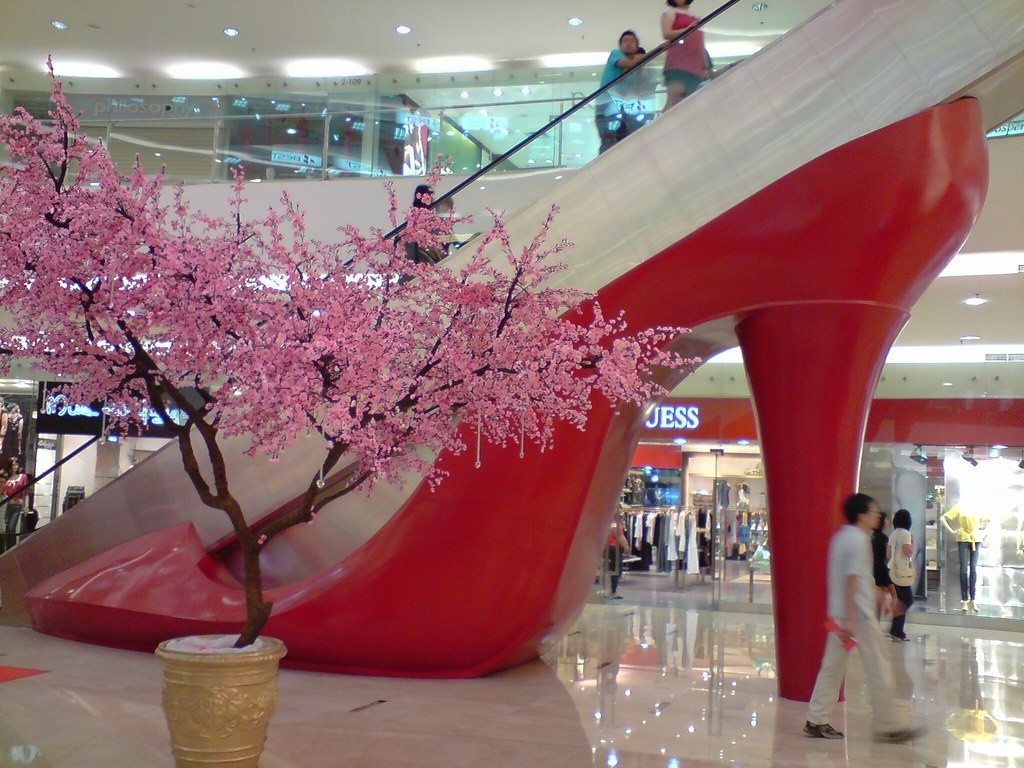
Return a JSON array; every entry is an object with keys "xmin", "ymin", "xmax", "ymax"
[
  {"xmin": 1018, "ymin": 450, "xmax": 1024, "ymax": 469},
  {"xmin": 962, "ymin": 446, "xmax": 980, "ymax": 467},
  {"xmin": 907, "ymin": 445, "xmax": 930, "ymax": 464}
]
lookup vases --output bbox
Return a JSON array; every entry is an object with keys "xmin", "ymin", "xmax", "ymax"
[{"xmin": 154, "ymin": 634, "xmax": 289, "ymax": 768}]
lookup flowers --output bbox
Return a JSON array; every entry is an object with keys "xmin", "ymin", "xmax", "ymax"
[{"xmin": 0, "ymin": 53, "xmax": 707, "ymax": 646}]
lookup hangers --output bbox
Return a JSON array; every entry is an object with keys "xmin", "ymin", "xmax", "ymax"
[{"xmin": 630, "ymin": 505, "xmax": 696, "ymax": 516}]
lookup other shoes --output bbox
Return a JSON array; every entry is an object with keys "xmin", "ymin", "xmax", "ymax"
[
  {"xmin": 612, "ymin": 593, "xmax": 623, "ymax": 599},
  {"xmin": 892, "ymin": 635, "xmax": 910, "ymax": 641}
]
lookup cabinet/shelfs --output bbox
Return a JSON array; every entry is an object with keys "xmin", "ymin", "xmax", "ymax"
[{"xmin": 688, "ymin": 472, "xmax": 941, "ymax": 581}]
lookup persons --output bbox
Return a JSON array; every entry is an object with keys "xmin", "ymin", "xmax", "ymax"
[
  {"xmin": 802, "ymin": 493, "xmax": 925, "ymax": 743},
  {"xmin": 0, "ymin": 395, "xmax": 24, "ymax": 457},
  {"xmin": 0, "ymin": 457, "xmax": 30, "ymax": 556},
  {"xmin": 607, "ymin": 502, "xmax": 631, "ymax": 600},
  {"xmin": 593, "ymin": 0, "xmax": 744, "ymax": 155},
  {"xmin": 940, "ymin": 492, "xmax": 992, "ymax": 613},
  {"xmin": 405, "ymin": 185, "xmax": 455, "ymax": 283}
]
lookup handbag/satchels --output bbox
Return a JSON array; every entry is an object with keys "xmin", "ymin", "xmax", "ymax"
[{"xmin": 889, "ymin": 562, "xmax": 915, "ymax": 586}]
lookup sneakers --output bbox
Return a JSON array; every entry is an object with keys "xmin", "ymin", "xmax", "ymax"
[
  {"xmin": 879, "ymin": 731, "xmax": 914, "ymax": 743},
  {"xmin": 802, "ymin": 720, "xmax": 844, "ymax": 739}
]
